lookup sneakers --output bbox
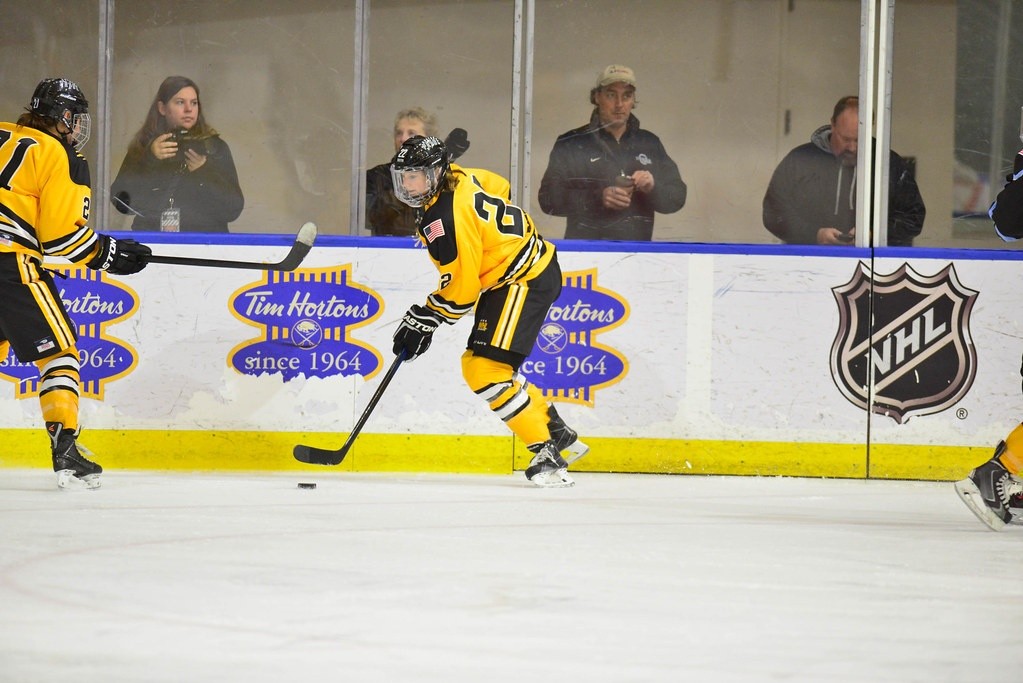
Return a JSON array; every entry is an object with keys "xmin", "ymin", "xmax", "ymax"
[
  {"xmin": 47, "ymin": 423, "xmax": 102, "ymax": 490},
  {"xmin": 524, "ymin": 440, "xmax": 576, "ymax": 489},
  {"xmin": 954, "ymin": 439, "xmax": 1023, "ymax": 532},
  {"xmin": 544, "ymin": 417, "xmax": 589, "ymax": 463}
]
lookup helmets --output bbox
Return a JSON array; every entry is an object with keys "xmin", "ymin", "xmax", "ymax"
[
  {"xmin": 30, "ymin": 78, "xmax": 91, "ymax": 152},
  {"xmin": 389, "ymin": 134, "xmax": 449, "ymax": 209}
]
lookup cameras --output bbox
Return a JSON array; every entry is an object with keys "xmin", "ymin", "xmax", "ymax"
[{"xmin": 164, "ymin": 129, "xmax": 207, "ymax": 162}]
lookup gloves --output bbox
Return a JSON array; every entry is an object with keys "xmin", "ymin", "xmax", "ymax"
[
  {"xmin": 393, "ymin": 304, "xmax": 442, "ymax": 364},
  {"xmin": 87, "ymin": 233, "xmax": 150, "ymax": 276}
]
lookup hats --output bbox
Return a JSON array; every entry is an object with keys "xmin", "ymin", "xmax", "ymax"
[{"xmin": 597, "ymin": 65, "xmax": 636, "ymax": 88}]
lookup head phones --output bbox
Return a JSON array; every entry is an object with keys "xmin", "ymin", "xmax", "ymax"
[{"xmin": 444, "ymin": 127, "xmax": 470, "ymax": 164}]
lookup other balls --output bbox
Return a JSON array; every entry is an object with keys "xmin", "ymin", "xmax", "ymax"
[{"xmin": 299, "ymin": 483, "xmax": 317, "ymax": 489}]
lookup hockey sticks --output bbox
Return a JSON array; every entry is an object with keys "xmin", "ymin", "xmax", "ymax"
[
  {"xmin": 152, "ymin": 218, "xmax": 320, "ymax": 274},
  {"xmin": 292, "ymin": 352, "xmax": 406, "ymax": 467}
]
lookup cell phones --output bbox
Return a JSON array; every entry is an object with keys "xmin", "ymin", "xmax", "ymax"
[{"xmin": 839, "ymin": 235, "xmax": 854, "ymax": 242}]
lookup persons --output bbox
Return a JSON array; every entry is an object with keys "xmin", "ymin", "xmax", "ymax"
[
  {"xmin": 538, "ymin": 64, "xmax": 687, "ymax": 243},
  {"xmin": 365, "ymin": 106, "xmax": 441, "ymax": 237},
  {"xmin": 392, "ymin": 134, "xmax": 589, "ymax": 488},
  {"xmin": 762, "ymin": 95, "xmax": 926, "ymax": 248},
  {"xmin": 110, "ymin": 76, "xmax": 243, "ymax": 232},
  {"xmin": 1, "ymin": 78, "xmax": 151, "ymax": 489},
  {"xmin": 955, "ymin": 142, "xmax": 1023, "ymax": 531}
]
